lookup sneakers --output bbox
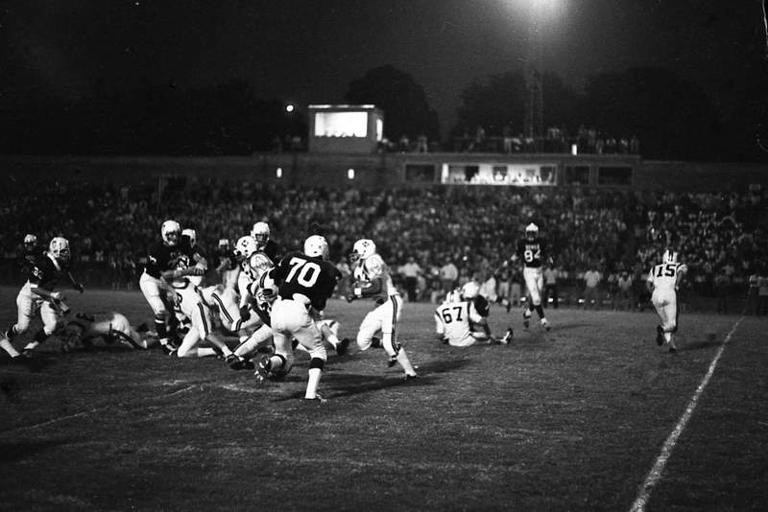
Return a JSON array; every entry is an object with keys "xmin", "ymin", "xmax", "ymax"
[
  {"xmin": 8, "ymin": 348, "xmax": 33, "ymax": 362},
  {"xmin": 542, "ymin": 321, "xmax": 553, "ymax": 331},
  {"xmin": 300, "ymin": 393, "xmax": 328, "ymax": 407},
  {"xmin": 387, "ymin": 341, "xmax": 402, "ymax": 368},
  {"xmin": 141, "ymin": 322, "xmax": 149, "ymax": 332},
  {"xmin": 254, "ymin": 354, "xmax": 271, "ymax": 385},
  {"xmin": 501, "ymin": 327, "xmax": 513, "ymax": 345},
  {"xmin": 335, "ymin": 337, "xmax": 350, "ymax": 357},
  {"xmin": 399, "ymin": 374, "xmax": 417, "ymax": 383},
  {"xmin": 224, "ymin": 353, "xmax": 248, "ymax": 371},
  {"xmin": 522, "ymin": 311, "xmax": 532, "ymax": 329},
  {"xmin": 158, "ymin": 337, "xmax": 177, "ymax": 356},
  {"xmin": 506, "ymin": 301, "xmax": 511, "ymax": 313},
  {"xmin": 669, "ymin": 347, "xmax": 681, "ymax": 354},
  {"xmin": 655, "ymin": 325, "xmax": 664, "ymax": 346}
]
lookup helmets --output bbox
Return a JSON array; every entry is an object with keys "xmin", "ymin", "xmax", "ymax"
[
  {"xmin": 462, "ymin": 281, "xmax": 480, "ymax": 300},
  {"xmin": 525, "ymin": 223, "xmax": 539, "ymax": 238},
  {"xmin": 249, "ymin": 221, "xmax": 270, "ymax": 251},
  {"xmin": 352, "ymin": 238, "xmax": 376, "ymax": 259},
  {"xmin": 49, "ymin": 236, "xmax": 71, "ymax": 261},
  {"xmin": 23, "ymin": 233, "xmax": 37, "ymax": 244},
  {"xmin": 303, "ymin": 234, "xmax": 330, "ymax": 263},
  {"xmin": 234, "ymin": 235, "xmax": 258, "ymax": 260},
  {"xmin": 181, "ymin": 228, "xmax": 197, "ymax": 249},
  {"xmin": 662, "ymin": 249, "xmax": 678, "ymax": 265},
  {"xmin": 161, "ymin": 219, "xmax": 183, "ymax": 247}
]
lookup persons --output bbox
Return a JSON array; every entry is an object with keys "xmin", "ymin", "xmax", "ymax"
[
  {"xmin": 379, "ymin": 120, "xmax": 638, "ymax": 155},
  {"xmin": 0, "ymin": 236, "xmax": 85, "ymax": 360},
  {"xmin": 516, "ymin": 222, "xmax": 551, "ymax": 332},
  {"xmin": 2, "ymin": 178, "xmax": 765, "ymax": 315},
  {"xmin": 645, "ymin": 249, "xmax": 687, "ymax": 352},
  {"xmin": 58, "ymin": 214, "xmax": 513, "ymax": 402}
]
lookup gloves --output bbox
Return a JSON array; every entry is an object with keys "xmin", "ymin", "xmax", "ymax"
[
  {"xmin": 72, "ymin": 281, "xmax": 84, "ymax": 293},
  {"xmin": 264, "ymin": 284, "xmax": 279, "ymax": 302}
]
